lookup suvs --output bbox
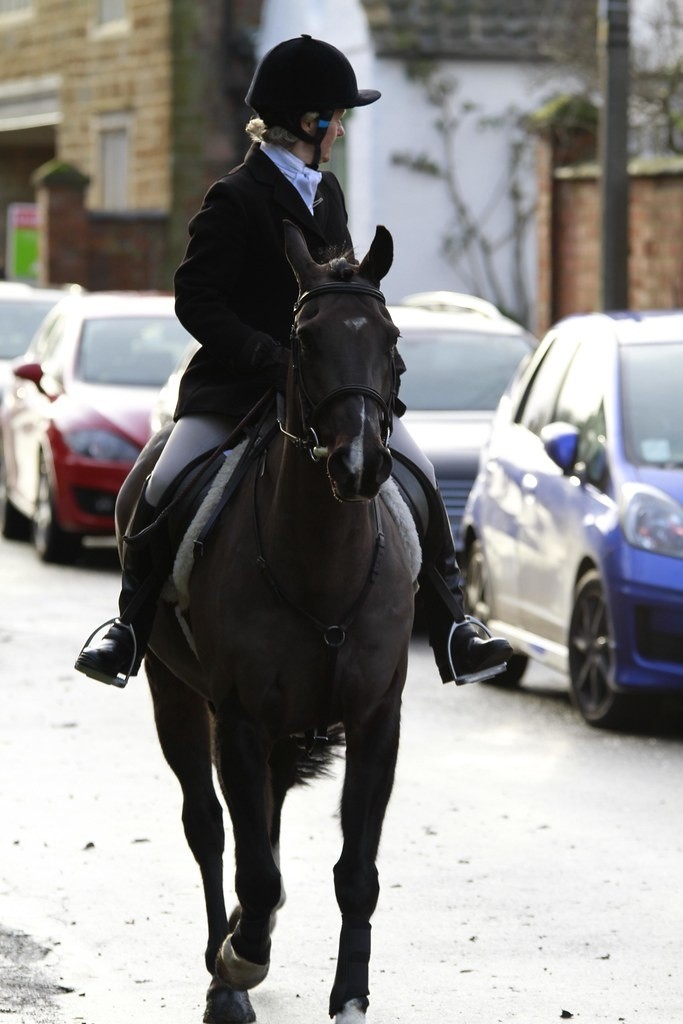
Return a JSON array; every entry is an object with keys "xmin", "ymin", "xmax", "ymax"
[{"xmin": 150, "ymin": 290, "xmax": 543, "ymax": 580}]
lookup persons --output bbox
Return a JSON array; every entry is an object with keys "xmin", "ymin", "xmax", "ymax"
[{"xmin": 73, "ymin": 33, "xmax": 515, "ymax": 690}]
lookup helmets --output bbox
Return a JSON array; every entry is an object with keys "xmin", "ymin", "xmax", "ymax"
[{"xmin": 245, "ymin": 34, "xmax": 381, "ymax": 135}]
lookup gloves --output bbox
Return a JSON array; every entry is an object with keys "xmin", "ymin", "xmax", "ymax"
[{"xmin": 264, "ymin": 339, "xmax": 292, "ymax": 380}]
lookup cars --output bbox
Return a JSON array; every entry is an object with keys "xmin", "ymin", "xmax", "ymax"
[
  {"xmin": 455, "ymin": 306, "xmax": 682, "ymax": 730},
  {"xmin": 1, "ymin": 288, "xmax": 199, "ymax": 564},
  {"xmin": 1, "ymin": 282, "xmax": 88, "ymax": 380}
]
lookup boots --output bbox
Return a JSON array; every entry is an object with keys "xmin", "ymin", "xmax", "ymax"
[
  {"xmin": 417, "ymin": 481, "xmax": 512, "ymax": 683},
  {"xmin": 76, "ymin": 476, "xmax": 166, "ymax": 678}
]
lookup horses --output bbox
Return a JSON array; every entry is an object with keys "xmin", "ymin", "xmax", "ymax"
[{"xmin": 109, "ymin": 215, "xmax": 428, "ymax": 1024}]
